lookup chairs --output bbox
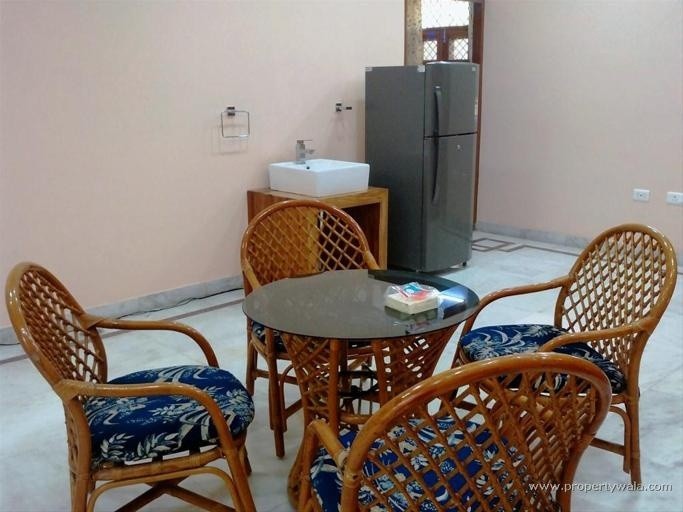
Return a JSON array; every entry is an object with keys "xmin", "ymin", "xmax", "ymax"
[
  {"xmin": 439, "ymin": 220, "xmax": 678, "ymax": 486},
  {"xmin": 240, "ymin": 198, "xmax": 383, "ymax": 459},
  {"xmin": 296, "ymin": 350, "xmax": 613, "ymax": 512},
  {"xmin": 5, "ymin": 261, "xmax": 260, "ymax": 512}
]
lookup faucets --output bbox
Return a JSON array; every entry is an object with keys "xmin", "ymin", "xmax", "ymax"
[{"xmin": 296, "ymin": 139, "xmax": 314, "ymax": 163}]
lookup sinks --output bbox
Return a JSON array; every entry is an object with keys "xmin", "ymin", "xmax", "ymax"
[{"xmin": 269, "ymin": 159, "xmax": 370, "ymax": 197}]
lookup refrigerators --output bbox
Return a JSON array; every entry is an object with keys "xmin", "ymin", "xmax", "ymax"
[{"xmin": 364, "ymin": 62, "xmax": 482, "ymax": 275}]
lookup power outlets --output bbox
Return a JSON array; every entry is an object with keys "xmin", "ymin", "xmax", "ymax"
[
  {"xmin": 633, "ymin": 190, "xmax": 650, "ymax": 203},
  {"xmin": 666, "ymin": 191, "xmax": 683, "ymax": 204}
]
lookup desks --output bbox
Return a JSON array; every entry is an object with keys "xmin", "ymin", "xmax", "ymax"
[{"xmin": 241, "ymin": 269, "xmax": 481, "ymax": 511}]
